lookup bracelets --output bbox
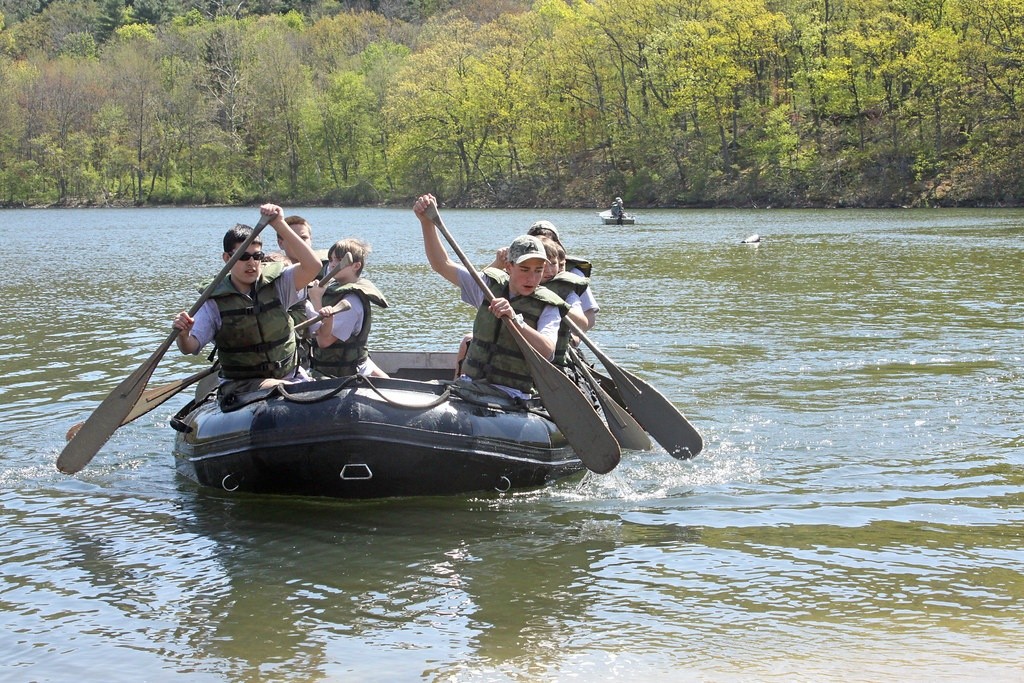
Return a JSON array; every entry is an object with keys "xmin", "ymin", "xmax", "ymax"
[{"xmin": 512, "ymin": 314, "xmax": 523, "ymax": 323}]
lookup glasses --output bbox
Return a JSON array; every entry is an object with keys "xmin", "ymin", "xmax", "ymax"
[{"xmin": 229, "ymin": 251, "xmax": 264, "ymax": 261}]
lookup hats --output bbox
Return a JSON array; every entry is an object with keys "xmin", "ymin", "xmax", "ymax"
[
  {"xmin": 509, "ymin": 235, "xmax": 551, "ymax": 264},
  {"xmin": 527, "ymin": 220, "xmax": 566, "ymax": 253}
]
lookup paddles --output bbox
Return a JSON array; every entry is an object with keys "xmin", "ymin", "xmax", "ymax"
[
  {"xmin": 506, "ymin": 257, "xmax": 704, "ymax": 461},
  {"xmin": 63, "ymin": 297, "xmax": 353, "ymax": 443},
  {"xmin": 428, "ymin": 199, "xmax": 622, "ymax": 474},
  {"xmin": 568, "ymin": 337, "xmax": 654, "ymax": 452},
  {"xmin": 54, "ymin": 210, "xmax": 276, "ymax": 477},
  {"xmin": 318, "ymin": 251, "xmax": 354, "ymax": 287}
]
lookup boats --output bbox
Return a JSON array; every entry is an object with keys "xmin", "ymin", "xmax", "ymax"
[
  {"xmin": 599, "ymin": 209, "xmax": 635, "ymax": 225},
  {"xmin": 170, "ymin": 352, "xmax": 600, "ymax": 499}
]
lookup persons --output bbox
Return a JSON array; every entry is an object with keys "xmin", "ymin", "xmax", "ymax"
[
  {"xmin": 611, "ymin": 197, "xmax": 628, "ymax": 218},
  {"xmin": 172, "ymin": 203, "xmax": 391, "ymax": 393},
  {"xmin": 413, "ymin": 194, "xmax": 599, "ymax": 394}
]
